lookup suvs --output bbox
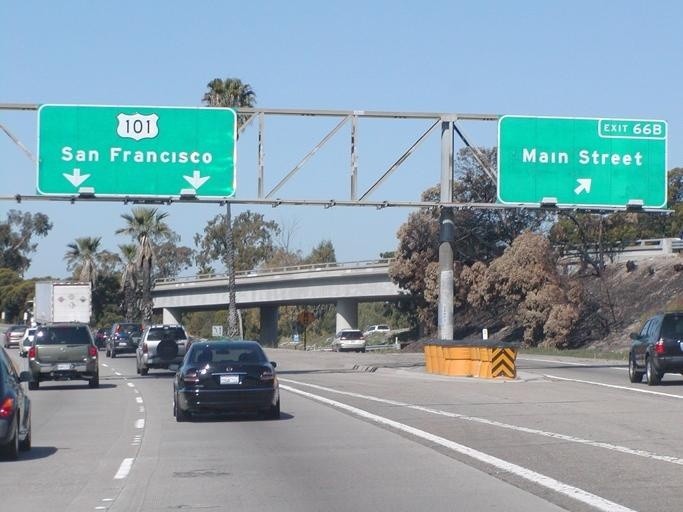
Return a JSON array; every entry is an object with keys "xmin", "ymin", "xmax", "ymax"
[
  {"xmin": 628, "ymin": 310, "xmax": 683, "ymax": 385},
  {"xmin": 135, "ymin": 325, "xmax": 193, "ymax": 376},
  {"xmin": 106, "ymin": 322, "xmax": 144, "ymax": 357},
  {"xmin": 28, "ymin": 324, "xmax": 99, "ymax": 390}
]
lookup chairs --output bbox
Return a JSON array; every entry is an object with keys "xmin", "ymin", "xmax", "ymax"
[
  {"xmin": 197, "ymin": 354, "xmax": 209, "ymax": 362},
  {"xmin": 238, "ymin": 353, "xmax": 249, "ymax": 362}
]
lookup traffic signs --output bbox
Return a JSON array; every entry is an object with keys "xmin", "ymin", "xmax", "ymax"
[
  {"xmin": 37, "ymin": 104, "xmax": 238, "ymax": 201},
  {"xmin": 495, "ymin": 116, "xmax": 669, "ymax": 213}
]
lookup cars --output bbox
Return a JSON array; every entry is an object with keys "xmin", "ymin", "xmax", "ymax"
[
  {"xmin": 361, "ymin": 324, "xmax": 390, "ymax": 336},
  {"xmin": 94, "ymin": 327, "xmax": 112, "ymax": 350},
  {"xmin": 0, "ymin": 345, "xmax": 31, "ymax": 461},
  {"xmin": 3, "ymin": 325, "xmax": 29, "ymax": 348},
  {"xmin": 20, "ymin": 328, "xmax": 37, "ymax": 358},
  {"xmin": 331, "ymin": 329, "xmax": 366, "ymax": 353},
  {"xmin": 173, "ymin": 341, "xmax": 281, "ymax": 422}
]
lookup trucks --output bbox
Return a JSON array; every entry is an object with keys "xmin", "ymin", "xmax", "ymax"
[{"xmin": 23, "ymin": 281, "xmax": 93, "ymax": 329}]
